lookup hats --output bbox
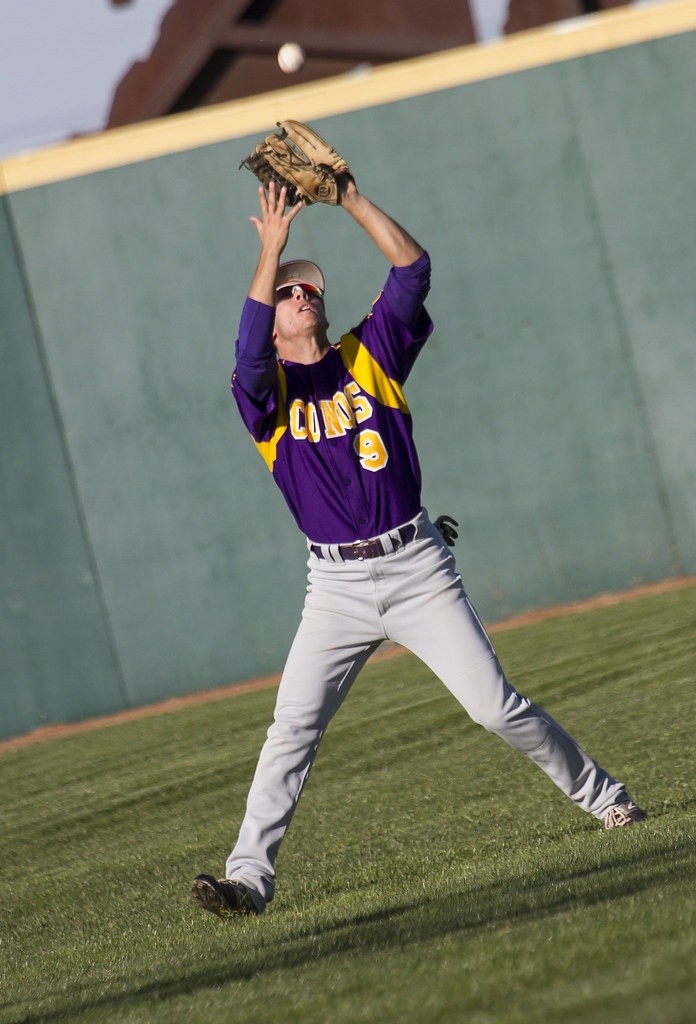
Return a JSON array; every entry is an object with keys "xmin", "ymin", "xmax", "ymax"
[{"xmin": 276, "ymin": 260, "xmax": 325, "ymax": 292}]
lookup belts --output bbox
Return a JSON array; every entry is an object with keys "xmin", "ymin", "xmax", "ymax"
[{"xmin": 311, "ymin": 525, "xmax": 415, "ymax": 561}]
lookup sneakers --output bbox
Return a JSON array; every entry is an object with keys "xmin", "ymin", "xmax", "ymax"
[
  {"xmin": 192, "ymin": 875, "xmax": 257, "ymax": 919},
  {"xmin": 603, "ymin": 800, "xmax": 648, "ymax": 828}
]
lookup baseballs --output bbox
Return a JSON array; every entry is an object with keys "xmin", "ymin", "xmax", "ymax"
[{"xmin": 279, "ymin": 40, "xmax": 306, "ymax": 75}]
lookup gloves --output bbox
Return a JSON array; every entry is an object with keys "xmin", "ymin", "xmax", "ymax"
[{"xmin": 432, "ymin": 515, "xmax": 459, "ymax": 547}]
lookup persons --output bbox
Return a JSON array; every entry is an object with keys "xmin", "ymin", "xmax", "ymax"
[{"xmin": 185, "ymin": 121, "xmax": 647, "ymax": 919}]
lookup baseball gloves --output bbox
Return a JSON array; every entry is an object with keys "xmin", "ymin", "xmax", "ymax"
[{"xmin": 247, "ymin": 118, "xmax": 356, "ymax": 207}]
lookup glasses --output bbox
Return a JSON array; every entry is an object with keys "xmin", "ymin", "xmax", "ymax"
[{"xmin": 276, "ymin": 284, "xmax": 324, "ymax": 304}]
